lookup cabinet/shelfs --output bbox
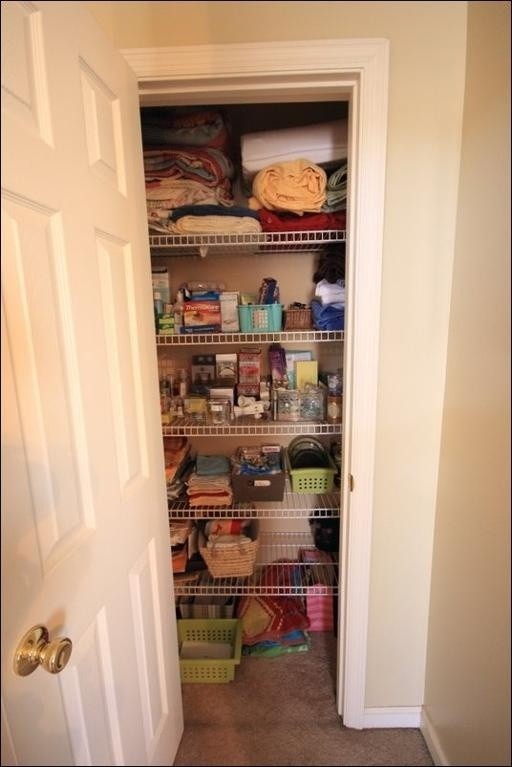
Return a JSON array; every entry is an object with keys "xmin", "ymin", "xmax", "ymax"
[{"xmin": 141, "ymin": 232, "xmax": 350, "ymax": 596}]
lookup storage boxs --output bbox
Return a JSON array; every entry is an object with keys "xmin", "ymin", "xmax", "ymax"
[{"xmin": 231, "ymin": 455, "xmax": 286, "ymax": 502}]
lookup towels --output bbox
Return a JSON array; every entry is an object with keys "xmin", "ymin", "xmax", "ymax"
[{"xmin": 185, "ymin": 454, "xmax": 232, "ymax": 505}]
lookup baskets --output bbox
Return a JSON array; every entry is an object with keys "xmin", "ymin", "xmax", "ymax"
[
  {"xmin": 283, "ymin": 308, "xmax": 312, "ymax": 330},
  {"xmin": 287, "ymin": 435, "xmax": 336, "ymax": 494},
  {"xmin": 198, "ymin": 519, "xmax": 259, "ymax": 578}
]
